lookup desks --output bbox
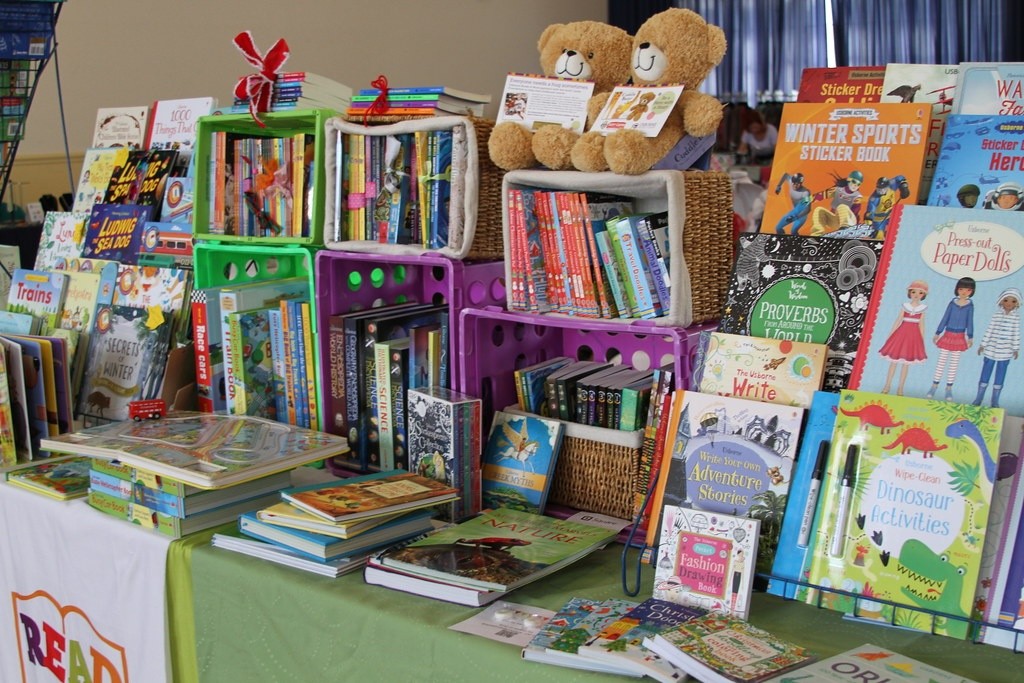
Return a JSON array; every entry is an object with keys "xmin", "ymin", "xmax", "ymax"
[{"xmin": 0, "ymin": 419, "xmax": 1024, "ymax": 683}]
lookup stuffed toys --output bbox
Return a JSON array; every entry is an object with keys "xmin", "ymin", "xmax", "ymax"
[
  {"xmin": 570, "ymin": 8, "xmax": 728, "ymax": 172},
  {"xmin": 486, "ymin": 21, "xmax": 640, "ymax": 171}
]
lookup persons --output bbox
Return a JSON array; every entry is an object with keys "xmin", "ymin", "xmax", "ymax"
[{"xmin": 737, "ymin": 110, "xmax": 778, "ymax": 167}]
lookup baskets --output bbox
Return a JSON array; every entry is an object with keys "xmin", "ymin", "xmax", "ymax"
[
  {"xmin": 506, "ymin": 404, "xmax": 643, "ymax": 520},
  {"xmin": 323, "ymin": 116, "xmax": 508, "ymax": 262},
  {"xmin": 501, "ymin": 166, "xmax": 734, "ymax": 326}
]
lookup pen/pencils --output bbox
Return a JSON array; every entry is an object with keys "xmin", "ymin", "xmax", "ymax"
[
  {"xmin": 796, "ymin": 439, "xmax": 830, "ymax": 546},
  {"xmin": 830, "ymin": 444, "xmax": 857, "ymax": 556}
]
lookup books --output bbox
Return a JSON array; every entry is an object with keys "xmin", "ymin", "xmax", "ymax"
[{"xmin": 1, "ymin": 60, "xmax": 1024, "ymax": 683}]
